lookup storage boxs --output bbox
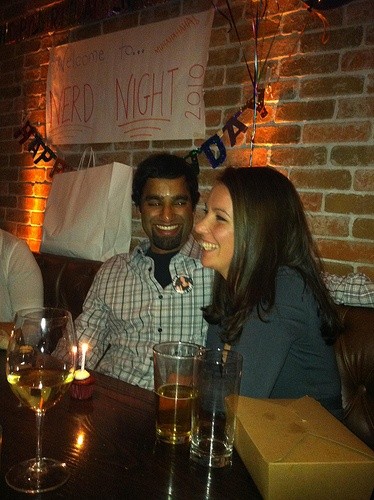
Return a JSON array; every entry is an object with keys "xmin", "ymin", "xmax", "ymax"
[{"xmin": 224, "ymin": 394, "xmax": 374, "ymax": 500}]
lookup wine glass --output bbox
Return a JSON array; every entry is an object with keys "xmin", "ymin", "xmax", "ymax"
[{"xmin": 3, "ymin": 307, "xmax": 74, "ymax": 495}]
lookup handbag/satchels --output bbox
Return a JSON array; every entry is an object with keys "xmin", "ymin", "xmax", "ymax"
[{"xmin": 39, "ymin": 146, "xmax": 133, "ymax": 262}]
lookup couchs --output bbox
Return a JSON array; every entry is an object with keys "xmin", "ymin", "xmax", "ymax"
[{"xmin": 31, "ymin": 251, "xmax": 374, "ymax": 450}]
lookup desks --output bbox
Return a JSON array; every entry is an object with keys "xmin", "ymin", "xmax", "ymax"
[{"xmin": 0, "ymin": 347, "xmax": 374, "ymax": 500}]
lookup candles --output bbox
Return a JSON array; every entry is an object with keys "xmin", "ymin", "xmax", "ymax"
[{"xmin": 80, "ymin": 343, "xmax": 90, "ymax": 380}]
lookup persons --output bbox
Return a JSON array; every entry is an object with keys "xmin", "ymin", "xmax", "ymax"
[
  {"xmin": 0, "ymin": 228, "xmax": 44, "ymax": 352},
  {"xmin": 50, "ymin": 154, "xmax": 374, "ymax": 392},
  {"xmin": 194, "ymin": 165, "xmax": 343, "ymax": 423}
]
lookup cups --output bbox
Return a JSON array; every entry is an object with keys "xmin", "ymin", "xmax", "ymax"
[
  {"xmin": 149, "ymin": 339, "xmax": 208, "ymax": 446},
  {"xmin": 187, "ymin": 348, "xmax": 245, "ymax": 469}
]
